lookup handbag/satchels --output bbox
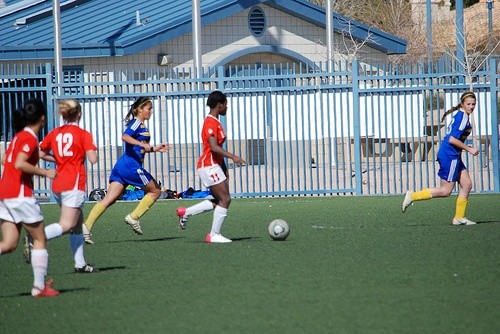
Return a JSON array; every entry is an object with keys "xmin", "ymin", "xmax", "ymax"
[{"xmin": 89, "ymin": 189, "xmax": 107, "ymax": 201}]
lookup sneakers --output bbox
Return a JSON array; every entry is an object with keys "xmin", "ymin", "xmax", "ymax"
[
  {"xmin": 124, "ymin": 213, "xmax": 143, "ymax": 235},
  {"xmin": 402, "ymin": 190, "xmax": 414, "ymax": 214},
  {"xmin": 31, "ymin": 278, "xmax": 59, "ymax": 297},
  {"xmin": 206, "ymin": 232, "xmax": 232, "ymax": 243},
  {"xmin": 23, "ymin": 234, "xmax": 33, "ymax": 263},
  {"xmin": 452, "ymin": 217, "xmax": 476, "ymax": 225},
  {"xmin": 75, "ymin": 265, "xmax": 99, "ymax": 273},
  {"xmin": 176, "ymin": 207, "xmax": 192, "ymax": 230},
  {"xmin": 82, "ymin": 223, "xmax": 94, "ymax": 245}
]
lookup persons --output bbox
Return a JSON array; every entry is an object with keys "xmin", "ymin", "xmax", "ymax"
[
  {"xmin": 23, "ymin": 99, "xmax": 101, "ymax": 272},
  {"xmin": 0, "ymin": 100, "xmax": 60, "ymax": 297},
  {"xmin": 82, "ymin": 96, "xmax": 171, "ymax": 245},
  {"xmin": 401, "ymin": 92, "xmax": 481, "ymax": 225},
  {"xmin": 177, "ymin": 91, "xmax": 246, "ymax": 243}
]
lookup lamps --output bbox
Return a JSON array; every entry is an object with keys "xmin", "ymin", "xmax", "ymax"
[{"xmin": 157, "ymin": 53, "xmax": 173, "ymax": 67}]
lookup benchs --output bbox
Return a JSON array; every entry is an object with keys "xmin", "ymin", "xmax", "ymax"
[{"xmin": 350, "ymin": 134, "xmax": 492, "ymax": 164}]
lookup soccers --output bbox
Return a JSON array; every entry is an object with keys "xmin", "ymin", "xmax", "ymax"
[{"xmin": 268, "ymin": 219, "xmax": 290, "ymax": 241}]
